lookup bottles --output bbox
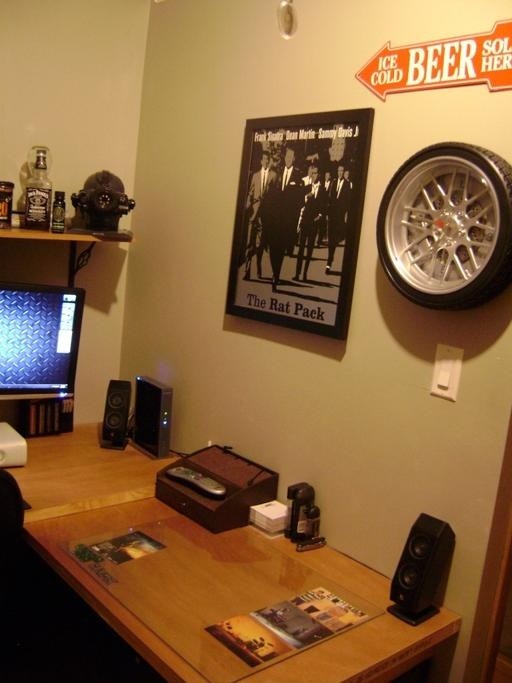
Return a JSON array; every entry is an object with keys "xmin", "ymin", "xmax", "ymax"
[
  {"xmin": 51, "ymin": 191, "xmax": 65, "ymax": 233},
  {"xmin": 0, "ymin": 182, "xmax": 14, "ymax": 228},
  {"xmin": 24, "ymin": 149, "xmax": 52, "ymax": 230}
]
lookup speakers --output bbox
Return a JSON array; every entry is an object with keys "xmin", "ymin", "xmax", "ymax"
[
  {"xmin": 383, "ymin": 512, "xmax": 457, "ymax": 626},
  {"xmin": 99, "ymin": 378, "xmax": 131, "ymax": 451}
]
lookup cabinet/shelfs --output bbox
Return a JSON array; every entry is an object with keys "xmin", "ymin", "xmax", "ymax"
[{"xmin": 0, "ymin": 222, "xmax": 133, "ymax": 434}]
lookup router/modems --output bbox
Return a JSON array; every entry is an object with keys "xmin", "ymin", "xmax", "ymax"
[{"xmin": 130, "ymin": 374, "xmax": 174, "ymax": 462}]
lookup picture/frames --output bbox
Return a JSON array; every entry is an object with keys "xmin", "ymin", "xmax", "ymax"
[{"xmin": 224, "ymin": 107, "xmax": 374, "ymax": 340}]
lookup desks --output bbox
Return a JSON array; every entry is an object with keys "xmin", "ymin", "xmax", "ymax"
[
  {"xmin": 24, "ymin": 498, "xmax": 462, "ymax": 682},
  {"xmin": 1, "ymin": 421, "xmax": 183, "ymax": 523}
]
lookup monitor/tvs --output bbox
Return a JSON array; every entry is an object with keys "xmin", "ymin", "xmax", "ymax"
[{"xmin": 0, "ymin": 278, "xmax": 86, "ymax": 401}]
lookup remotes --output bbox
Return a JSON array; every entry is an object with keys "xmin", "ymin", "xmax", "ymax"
[{"xmin": 167, "ymin": 464, "xmax": 226, "ymax": 499}]
